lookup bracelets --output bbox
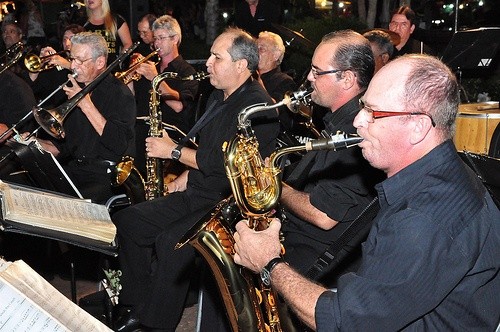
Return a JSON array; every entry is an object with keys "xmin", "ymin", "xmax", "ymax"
[{"xmin": 173, "ymin": 181, "xmax": 180, "ymax": 192}]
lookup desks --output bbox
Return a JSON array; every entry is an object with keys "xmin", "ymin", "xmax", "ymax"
[{"xmin": 455, "ymin": 101, "xmax": 500, "ymax": 155}]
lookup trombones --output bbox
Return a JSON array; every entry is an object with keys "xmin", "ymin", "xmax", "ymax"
[
  {"xmin": 0, "ymin": 42, "xmax": 140, "ymax": 166},
  {"xmin": 0, "ymin": 34, "xmax": 43, "ymax": 75}
]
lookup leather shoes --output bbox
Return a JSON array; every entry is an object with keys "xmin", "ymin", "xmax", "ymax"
[
  {"xmin": 80, "ymin": 295, "xmax": 122, "ymax": 318},
  {"xmin": 113, "ymin": 310, "xmax": 141, "ymax": 332}
]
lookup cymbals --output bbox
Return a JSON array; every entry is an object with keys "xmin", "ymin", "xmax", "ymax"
[
  {"xmin": 454, "ymin": 27, "xmax": 500, "ymax": 34},
  {"xmin": 272, "ymin": 21, "xmax": 320, "ymax": 49}
]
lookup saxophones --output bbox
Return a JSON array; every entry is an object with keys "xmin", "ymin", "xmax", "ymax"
[
  {"xmin": 180, "ymin": 131, "xmax": 374, "ymax": 331},
  {"xmin": 117, "ymin": 70, "xmax": 212, "ymax": 200},
  {"xmin": 236, "ymin": 88, "xmax": 314, "ymax": 153}
]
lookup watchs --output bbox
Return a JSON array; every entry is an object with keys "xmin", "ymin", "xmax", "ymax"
[
  {"xmin": 171, "ymin": 144, "xmax": 184, "ymax": 162},
  {"xmin": 259, "ymin": 256, "xmax": 289, "ymax": 286}
]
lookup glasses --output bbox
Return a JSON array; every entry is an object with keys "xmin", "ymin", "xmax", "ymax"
[
  {"xmin": 312, "ymin": 64, "xmax": 357, "ymax": 79},
  {"xmin": 69, "ymin": 55, "xmax": 101, "ymax": 65},
  {"xmin": 154, "ymin": 35, "xmax": 174, "ymax": 41},
  {"xmin": 358, "ymin": 99, "xmax": 436, "ymax": 128}
]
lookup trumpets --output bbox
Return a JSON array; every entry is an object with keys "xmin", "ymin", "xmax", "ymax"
[
  {"xmin": 23, "ymin": 49, "xmax": 75, "ymax": 72},
  {"xmin": 114, "ymin": 49, "xmax": 162, "ymax": 81}
]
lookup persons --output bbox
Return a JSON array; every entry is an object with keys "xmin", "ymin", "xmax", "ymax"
[
  {"xmin": 132, "ymin": 14, "xmax": 159, "ymax": 62},
  {"xmin": 30, "ymin": 25, "xmax": 86, "ymax": 109},
  {"xmin": 388, "ymin": 5, "xmax": 434, "ymax": 56},
  {"xmin": 0, "ymin": 1, "xmax": 45, "ymax": 142},
  {"xmin": 7, "ymin": 31, "xmax": 138, "ymax": 201},
  {"xmin": 234, "ymin": 0, "xmax": 277, "ymax": 36},
  {"xmin": 234, "ymin": 54, "xmax": 500, "ymax": 332},
  {"xmin": 363, "ymin": 30, "xmax": 393, "ymax": 74},
  {"xmin": 113, "ymin": 28, "xmax": 278, "ymax": 332},
  {"xmin": 262, "ymin": 29, "xmax": 386, "ymax": 277},
  {"xmin": 128, "ymin": 14, "xmax": 198, "ymax": 169},
  {"xmin": 256, "ymin": 32, "xmax": 296, "ymax": 104},
  {"xmin": 83, "ymin": 0, "xmax": 133, "ymax": 73}
]
